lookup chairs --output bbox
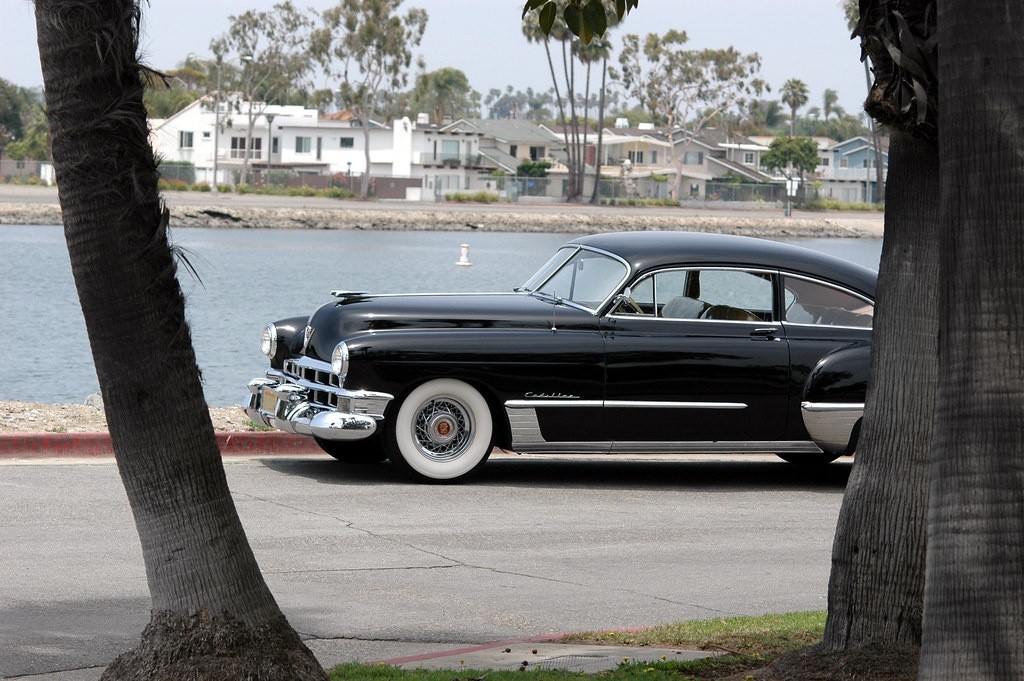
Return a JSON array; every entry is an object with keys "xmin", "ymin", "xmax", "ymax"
[
  {"xmin": 785, "ymin": 303, "xmax": 874, "ymax": 327},
  {"xmin": 658, "ymin": 295, "xmax": 763, "ymax": 321}
]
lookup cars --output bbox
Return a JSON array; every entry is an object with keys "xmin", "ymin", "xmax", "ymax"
[{"xmin": 242, "ymin": 231, "xmax": 877, "ymax": 488}]
